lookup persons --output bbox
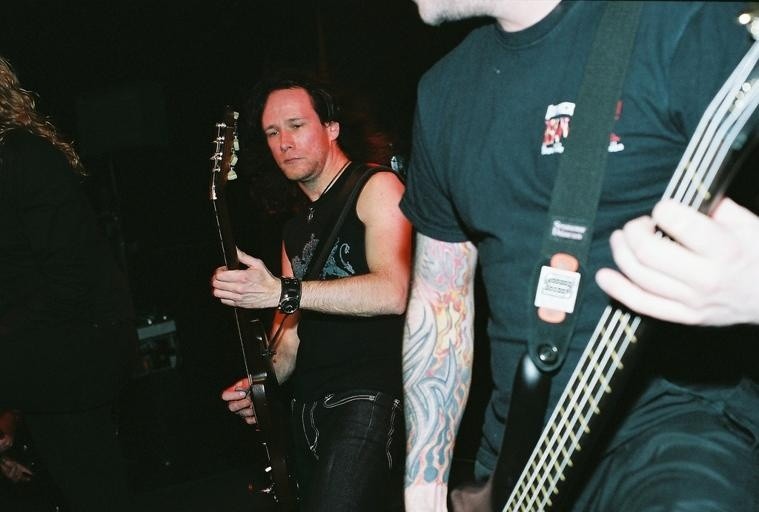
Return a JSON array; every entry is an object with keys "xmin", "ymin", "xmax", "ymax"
[
  {"xmin": 401, "ymin": 0, "xmax": 758, "ymax": 512},
  {"xmin": 211, "ymin": 80, "xmax": 412, "ymax": 512}
]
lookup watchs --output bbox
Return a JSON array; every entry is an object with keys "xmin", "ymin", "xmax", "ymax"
[{"xmin": 277, "ymin": 276, "xmax": 302, "ymax": 315}]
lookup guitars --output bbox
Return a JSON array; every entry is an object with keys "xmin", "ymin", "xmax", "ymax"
[
  {"xmin": 209, "ymin": 107, "xmax": 301, "ymax": 510},
  {"xmin": 492, "ymin": 9, "xmax": 759, "ymax": 512}
]
